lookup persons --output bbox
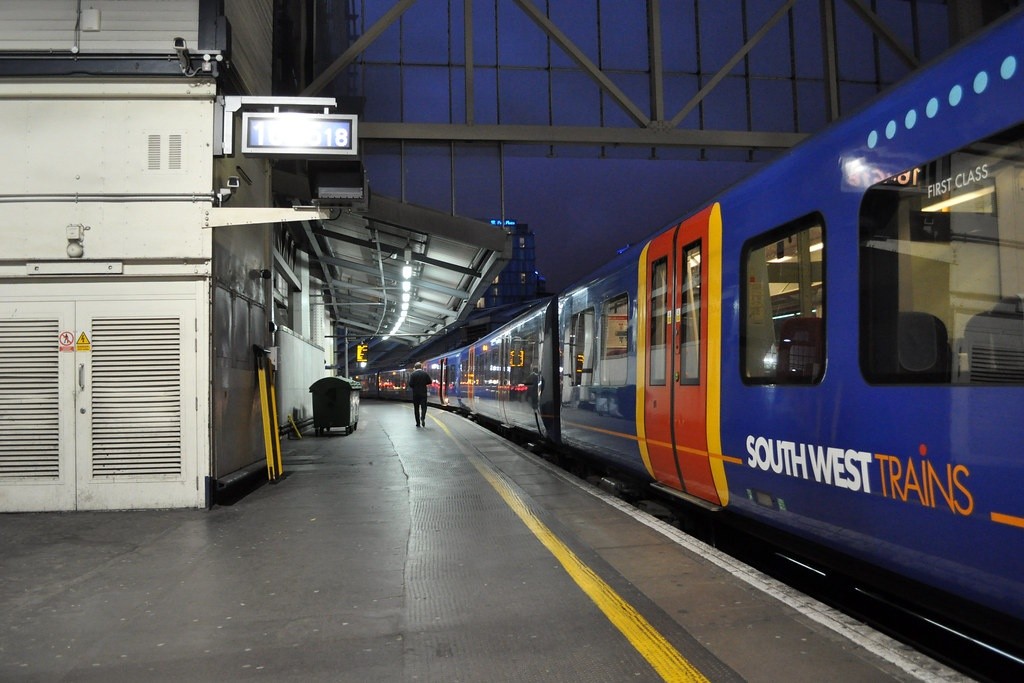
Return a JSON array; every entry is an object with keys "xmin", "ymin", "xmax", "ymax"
[
  {"xmin": 526, "ymin": 367, "xmax": 539, "ymax": 413},
  {"xmin": 409, "ymin": 363, "xmax": 432, "ymax": 426}
]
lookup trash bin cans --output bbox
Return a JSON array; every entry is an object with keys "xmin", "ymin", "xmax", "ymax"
[{"xmin": 308, "ymin": 376, "xmax": 362, "ymax": 436}]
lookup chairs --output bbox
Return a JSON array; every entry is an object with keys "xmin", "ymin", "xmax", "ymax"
[
  {"xmin": 892, "ymin": 308, "xmax": 953, "ymax": 384},
  {"xmin": 774, "ymin": 314, "xmax": 824, "ymax": 382}
]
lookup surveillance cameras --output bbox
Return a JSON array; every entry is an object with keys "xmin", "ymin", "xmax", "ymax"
[
  {"xmin": 227, "ymin": 176, "xmax": 240, "ymax": 190},
  {"xmin": 173, "ymin": 37, "xmax": 190, "ymax": 71}
]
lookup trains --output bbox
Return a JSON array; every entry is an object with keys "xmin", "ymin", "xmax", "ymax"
[{"xmin": 356, "ymin": 11, "xmax": 1024, "ymax": 677}]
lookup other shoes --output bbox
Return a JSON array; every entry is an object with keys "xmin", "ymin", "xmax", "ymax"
[
  {"xmin": 421, "ymin": 417, "xmax": 425, "ymax": 427},
  {"xmin": 416, "ymin": 423, "xmax": 420, "ymax": 426}
]
